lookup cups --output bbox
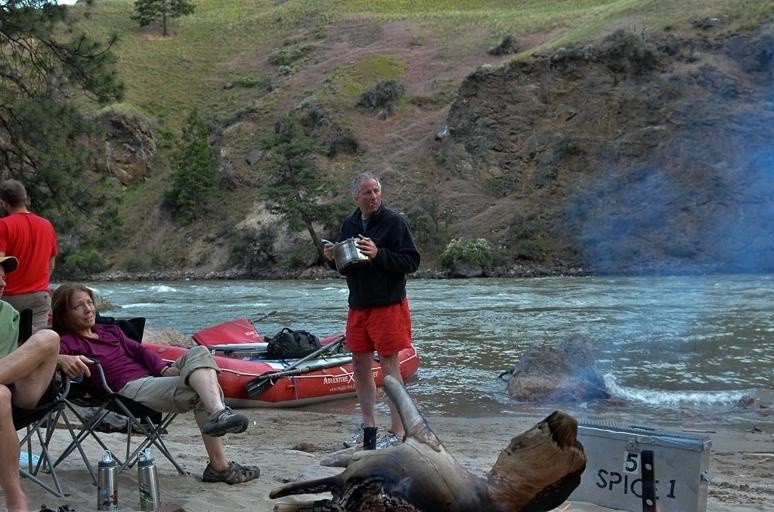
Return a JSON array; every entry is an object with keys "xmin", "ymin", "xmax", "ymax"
[{"xmin": 364, "ymin": 427, "xmax": 378, "ymax": 450}]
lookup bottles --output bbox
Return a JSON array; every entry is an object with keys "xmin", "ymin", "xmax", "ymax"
[
  {"xmin": 97, "ymin": 450, "xmax": 118, "ymax": 511},
  {"xmin": 136, "ymin": 451, "xmax": 160, "ymax": 512}
]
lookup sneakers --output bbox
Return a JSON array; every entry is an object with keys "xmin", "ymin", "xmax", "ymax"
[
  {"xmin": 343, "ymin": 423, "xmax": 380, "ymax": 447},
  {"xmin": 203, "ymin": 460, "xmax": 260, "ymax": 484},
  {"xmin": 203, "ymin": 406, "xmax": 248, "ymax": 437},
  {"xmin": 376, "ymin": 431, "xmax": 405, "ymax": 449}
]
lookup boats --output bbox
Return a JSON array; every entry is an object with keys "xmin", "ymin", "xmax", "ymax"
[{"xmin": 140, "ymin": 319, "xmax": 420, "ymax": 405}]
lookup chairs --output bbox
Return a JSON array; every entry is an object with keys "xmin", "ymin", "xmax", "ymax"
[{"xmin": 13, "ymin": 308, "xmax": 184, "ymax": 497}]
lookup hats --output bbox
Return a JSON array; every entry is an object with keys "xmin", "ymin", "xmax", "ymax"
[{"xmin": 0, "ymin": 256, "xmax": 19, "ymax": 273}]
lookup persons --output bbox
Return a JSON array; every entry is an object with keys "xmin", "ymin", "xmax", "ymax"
[
  {"xmin": 51, "ymin": 283, "xmax": 260, "ymax": 485},
  {"xmin": 323, "ymin": 173, "xmax": 421, "ymax": 452},
  {"xmin": 0, "ymin": 256, "xmax": 61, "ymax": 512},
  {"xmin": 0, "ymin": 179, "xmax": 58, "ymax": 427}
]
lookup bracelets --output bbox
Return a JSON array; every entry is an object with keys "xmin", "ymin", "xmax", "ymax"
[{"xmin": 160, "ymin": 365, "xmax": 168, "ymax": 375}]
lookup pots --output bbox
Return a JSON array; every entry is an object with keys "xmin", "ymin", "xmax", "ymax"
[{"xmin": 332, "ymin": 237, "xmax": 372, "ymax": 276}]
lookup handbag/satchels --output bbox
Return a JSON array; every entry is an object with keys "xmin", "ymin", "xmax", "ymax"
[{"xmin": 267, "ymin": 328, "xmax": 321, "ymax": 359}]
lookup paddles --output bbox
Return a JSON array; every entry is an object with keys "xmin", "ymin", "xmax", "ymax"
[{"xmin": 245, "ymin": 335, "xmax": 346, "ymax": 399}]
